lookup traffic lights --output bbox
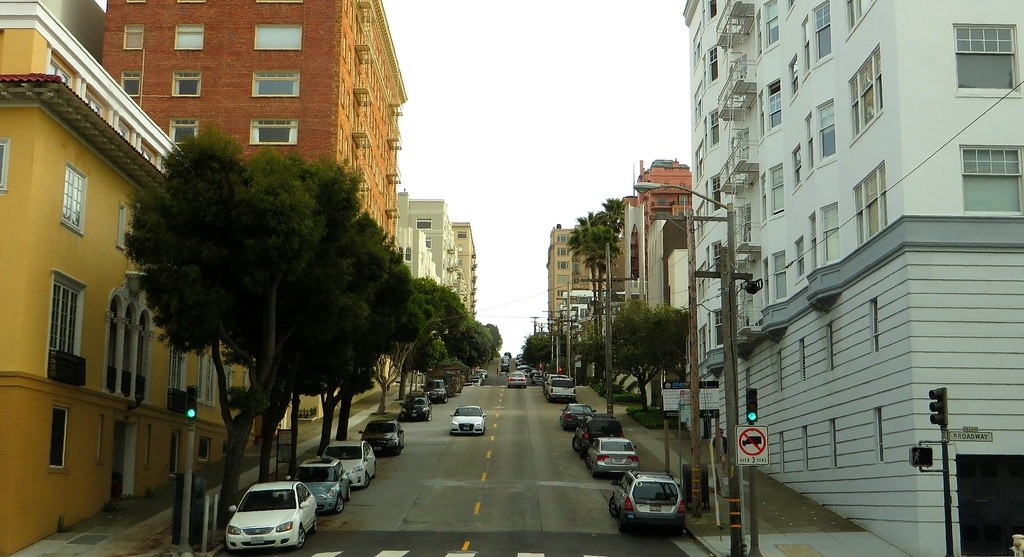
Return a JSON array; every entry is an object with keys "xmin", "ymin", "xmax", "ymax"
[
  {"xmin": 185, "ymin": 386, "xmax": 198, "ymax": 420},
  {"xmin": 745, "ymin": 388, "xmax": 758, "ymax": 423},
  {"xmin": 928, "ymin": 387, "xmax": 949, "ymax": 426}
]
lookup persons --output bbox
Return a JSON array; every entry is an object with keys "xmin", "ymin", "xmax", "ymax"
[{"xmin": 713, "ymin": 428, "xmax": 727, "ymax": 477}]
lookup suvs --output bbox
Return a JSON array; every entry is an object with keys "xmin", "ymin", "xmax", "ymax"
[
  {"xmin": 572, "ymin": 413, "xmax": 624, "ymax": 452},
  {"xmin": 609, "ymin": 469, "xmax": 685, "ymax": 535}
]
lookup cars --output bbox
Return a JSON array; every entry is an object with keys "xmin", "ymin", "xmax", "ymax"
[
  {"xmin": 225, "ymin": 481, "xmax": 318, "ymax": 550},
  {"xmin": 321, "ymin": 440, "xmax": 375, "ymax": 488},
  {"xmin": 421, "ymin": 379, "xmax": 448, "ymax": 403},
  {"xmin": 464, "ymin": 369, "xmax": 488, "ymax": 387},
  {"xmin": 584, "ymin": 436, "xmax": 640, "ymax": 478},
  {"xmin": 559, "ymin": 402, "xmax": 597, "ymax": 430},
  {"xmin": 358, "ymin": 419, "xmax": 405, "ymax": 457},
  {"xmin": 507, "ymin": 372, "xmax": 527, "ymax": 388},
  {"xmin": 542, "ymin": 374, "xmax": 576, "ymax": 403},
  {"xmin": 500, "ymin": 352, "xmax": 511, "ymax": 375},
  {"xmin": 399, "ymin": 395, "xmax": 432, "ymax": 421},
  {"xmin": 515, "ymin": 354, "xmax": 544, "ymax": 387},
  {"xmin": 284, "ymin": 456, "xmax": 351, "ymax": 514},
  {"xmin": 449, "ymin": 405, "xmax": 487, "ymax": 436}
]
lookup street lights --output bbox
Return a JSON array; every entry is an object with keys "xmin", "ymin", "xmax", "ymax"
[{"xmin": 633, "ymin": 180, "xmax": 743, "ymax": 557}]
